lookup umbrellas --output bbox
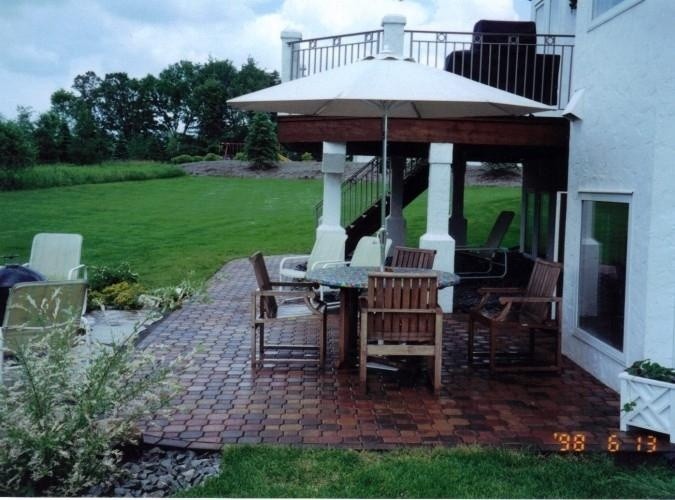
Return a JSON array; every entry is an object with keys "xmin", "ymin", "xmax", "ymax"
[{"xmin": 228, "ymin": 45, "xmax": 557, "ymax": 357}]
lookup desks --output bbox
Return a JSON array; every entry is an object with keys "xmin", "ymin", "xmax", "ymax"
[{"xmin": 303, "ymin": 264, "xmax": 463, "ymax": 373}]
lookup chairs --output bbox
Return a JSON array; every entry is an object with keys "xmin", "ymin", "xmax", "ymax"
[
  {"xmin": 20, "ymin": 233, "xmax": 87, "ymax": 316},
  {"xmin": 310, "ymin": 235, "xmax": 392, "ymax": 312},
  {"xmin": 451, "ymin": 208, "xmax": 516, "ymax": 280},
  {"xmin": 467, "ymin": 257, "xmax": 565, "ymax": 375},
  {"xmin": 387, "ymin": 245, "xmax": 439, "ymax": 270},
  {"xmin": 444, "ymin": 19, "xmax": 564, "ymax": 107},
  {"xmin": 247, "ymin": 249, "xmax": 329, "ymax": 374},
  {"xmin": 277, "ymin": 222, "xmax": 350, "ymax": 297},
  {"xmin": 356, "ymin": 271, "xmax": 445, "ymax": 394},
  {"xmin": 2, "ymin": 277, "xmax": 92, "ymax": 363}
]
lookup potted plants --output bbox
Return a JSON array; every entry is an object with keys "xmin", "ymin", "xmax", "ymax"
[{"xmin": 617, "ymin": 357, "xmax": 675, "ymax": 449}]
